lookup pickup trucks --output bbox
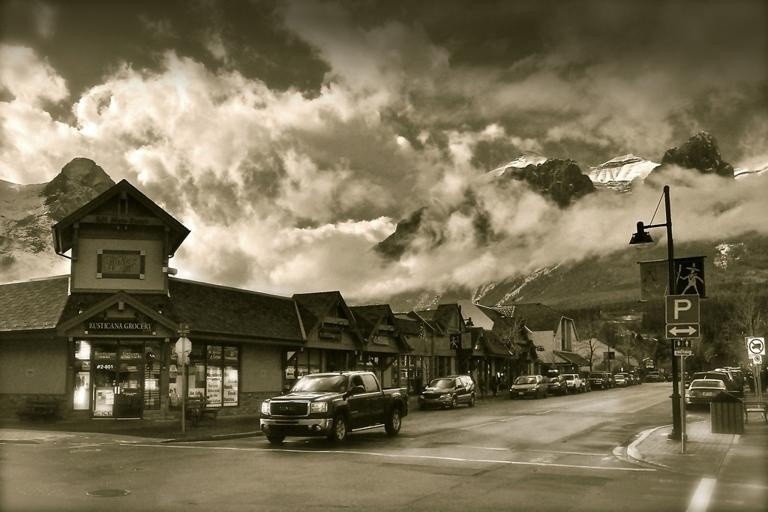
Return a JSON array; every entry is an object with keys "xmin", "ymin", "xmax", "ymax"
[{"xmin": 260, "ymin": 372, "xmax": 409, "ymax": 445}]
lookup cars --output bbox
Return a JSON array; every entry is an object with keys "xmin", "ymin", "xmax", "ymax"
[
  {"xmin": 685, "ymin": 366, "xmax": 743, "ymax": 409},
  {"xmin": 668, "ymin": 373, "xmax": 681, "ymax": 381},
  {"xmin": 646, "ymin": 370, "xmax": 665, "ymax": 382},
  {"xmin": 549, "ymin": 369, "xmax": 641, "ymax": 395}
]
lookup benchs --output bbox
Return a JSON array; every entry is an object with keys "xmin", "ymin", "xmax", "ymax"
[
  {"xmin": 187, "ymin": 396, "xmax": 221, "ymax": 426},
  {"xmin": 17, "ymin": 399, "xmax": 59, "ymax": 421},
  {"xmin": 743, "ymin": 401, "xmax": 767, "ymax": 423}
]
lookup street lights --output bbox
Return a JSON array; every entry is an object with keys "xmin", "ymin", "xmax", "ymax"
[{"xmin": 629, "ymin": 184, "xmax": 682, "ymax": 438}]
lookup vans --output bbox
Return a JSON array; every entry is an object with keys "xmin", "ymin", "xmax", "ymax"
[
  {"xmin": 509, "ymin": 375, "xmax": 547, "ymax": 399},
  {"xmin": 421, "ymin": 374, "xmax": 475, "ymax": 409}
]
